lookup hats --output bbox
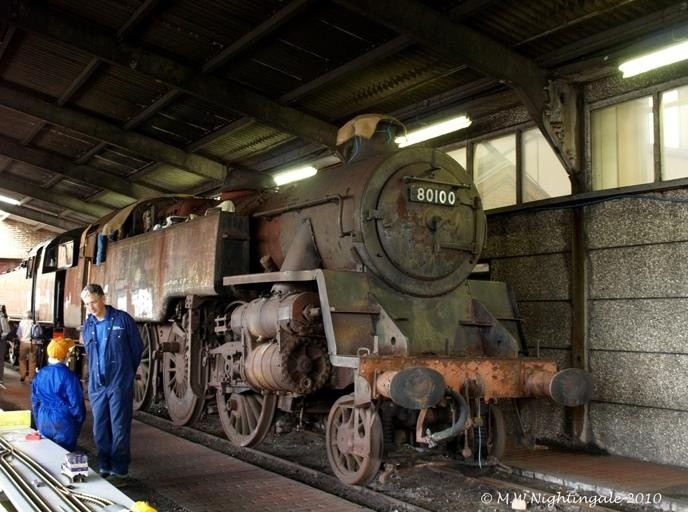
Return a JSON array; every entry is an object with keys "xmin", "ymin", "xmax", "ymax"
[{"xmin": 46, "ymin": 336, "xmax": 76, "ymax": 359}]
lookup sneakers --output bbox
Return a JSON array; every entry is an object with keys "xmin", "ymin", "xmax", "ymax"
[
  {"xmin": 20, "ymin": 378, "xmax": 25, "ymax": 384},
  {"xmin": 97, "ymin": 471, "xmax": 110, "ymax": 478},
  {"xmin": 104, "ymin": 475, "xmax": 128, "ymax": 487}
]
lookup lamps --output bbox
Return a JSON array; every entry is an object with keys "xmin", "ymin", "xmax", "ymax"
[{"xmin": 393, "ymin": 112, "xmax": 471, "ymax": 150}]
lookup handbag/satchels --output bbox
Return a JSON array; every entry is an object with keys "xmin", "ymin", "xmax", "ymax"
[{"xmin": 4, "ymin": 322, "xmax": 16, "ymax": 341}]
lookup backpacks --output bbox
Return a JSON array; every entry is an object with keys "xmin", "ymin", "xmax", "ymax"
[{"xmin": 30, "ymin": 322, "xmax": 43, "ymax": 339}]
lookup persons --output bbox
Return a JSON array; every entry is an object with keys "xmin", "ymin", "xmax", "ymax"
[
  {"xmin": 78, "ymin": 283, "xmax": 144, "ymax": 488},
  {"xmin": 30, "ymin": 335, "xmax": 86, "ymax": 452},
  {"xmin": 0, "ymin": 304, "xmax": 43, "ymax": 390}
]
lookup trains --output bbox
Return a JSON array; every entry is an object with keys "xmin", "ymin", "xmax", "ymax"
[{"xmin": 0, "ymin": 113, "xmax": 595, "ymax": 487}]
